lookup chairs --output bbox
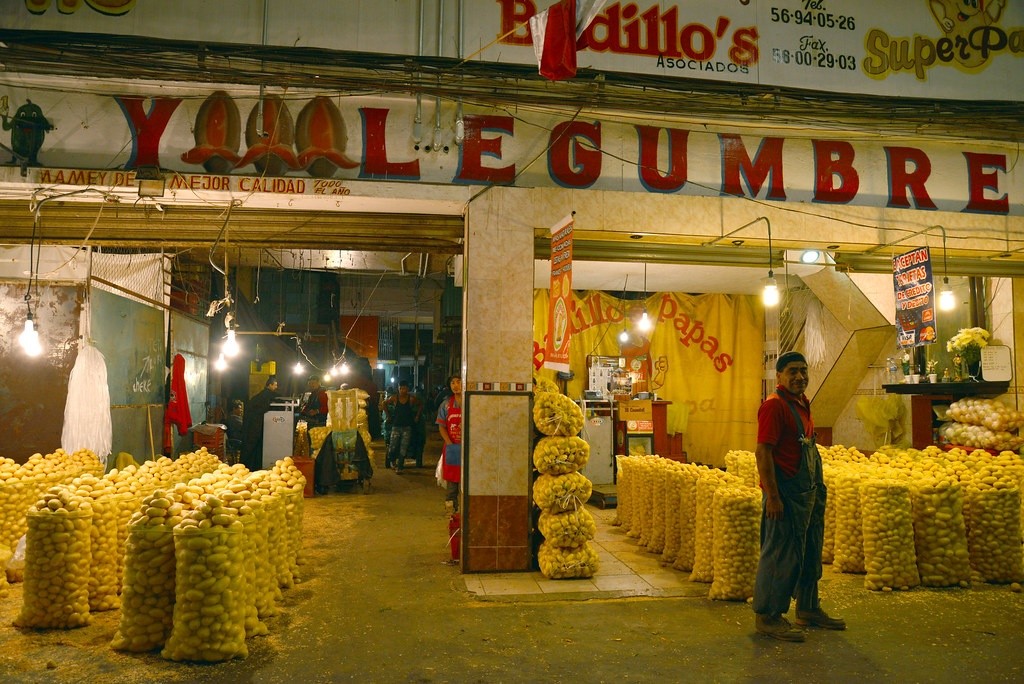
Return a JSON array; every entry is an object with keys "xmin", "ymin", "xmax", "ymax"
[{"xmin": 224, "ymin": 438, "xmax": 243, "ymax": 465}]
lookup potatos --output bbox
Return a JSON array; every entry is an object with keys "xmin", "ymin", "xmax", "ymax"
[
  {"xmin": 532, "ymin": 376, "xmax": 600, "ymax": 580},
  {"xmin": 612, "ymin": 444, "xmax": 1024, "ymax": 604},
  {"xmin": 295, "ymin": 388, "xmax": 375, "ymax": 472},
  {"xmin": 945, "ymin": 398, "xmax": 1024, "ymax": 451},
  {"xmin": 0, "ymin": 447, "xmax": 309, "ymax": 663}
]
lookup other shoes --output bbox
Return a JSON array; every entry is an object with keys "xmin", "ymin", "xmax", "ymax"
[
  {"xmin": 395, "ymin": 466, "xmax": 402, "ymax": 474},
  {"xmin": 755, "ymin": 613, "xmax": 805, "ymax": 641},
  {"xmin": 795, "ymin": 606, "xmax": 846, "ymax": 630},
  {"xmin": 390, "ymin": 458, "xmax": 396, "ymax": 469}
]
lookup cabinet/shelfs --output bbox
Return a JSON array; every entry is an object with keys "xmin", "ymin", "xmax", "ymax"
[
  {"xmin": 911, "ymin": 393, "xmax": 959, "ymax": 451},
  {"xmin": 574, "ymin": 397, "xmax": 673, "ymax": 460}
]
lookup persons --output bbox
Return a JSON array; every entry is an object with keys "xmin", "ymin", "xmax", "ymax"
[
  {"xmin": 229, "ymin": 399, "xmax": 243, "ymax": 440},
  {"xmin": 294, "ymin": 375, "xmax": 329, "ymax": 427},
  {"xmin": 434, "ymin": 375, "xmax": 462, "ymax": 519},
  {"xmin": 239, "ymin": 377, "xmax": 278, "ymax": 466},
  {"xmin": 382, "ymin": 380, "xmax": 423, "ymax": 474},
  {"xmin": 754, "ymin": 351, "xmax": 845, "ymax": 640},
  {"xmin": 326, "ymin": 383, "xmax": 350, "ymax": 427}
]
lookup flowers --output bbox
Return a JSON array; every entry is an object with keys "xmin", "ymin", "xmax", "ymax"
[{"xmin": 946, "ymin": 326, "xmax": 990, "ymax": 354}]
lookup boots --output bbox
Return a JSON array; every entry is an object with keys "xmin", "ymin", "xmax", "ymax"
[{"xmin": 444, "ymin": 499, "xmax": 455, "ymax": 518}]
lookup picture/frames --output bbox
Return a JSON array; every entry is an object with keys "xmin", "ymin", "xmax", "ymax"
[{"xmin": 624, "ymin": 433, "xmax": 654, "ymax": 456}]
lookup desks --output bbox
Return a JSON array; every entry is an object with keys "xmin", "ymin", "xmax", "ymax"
[{"xmin": 881, "ymin": 382, "xmax": 1012, "ymax": 397}]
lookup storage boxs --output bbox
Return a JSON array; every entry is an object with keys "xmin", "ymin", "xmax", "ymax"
[
  {"xmin": 194, "ymin": 427, "xmax": 224, "ymax": 463},
  {"xmin": 616, "ymin": 400, "xmax": 654, "ymax": 435},
  {"xmin": 251, "ymin": 361, "xmax": 276, "ymax": 376}
]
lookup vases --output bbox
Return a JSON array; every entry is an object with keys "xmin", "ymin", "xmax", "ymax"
[{"xmin": 960, "ymin": 347, "xmax": 982, "ymax": 380}]
